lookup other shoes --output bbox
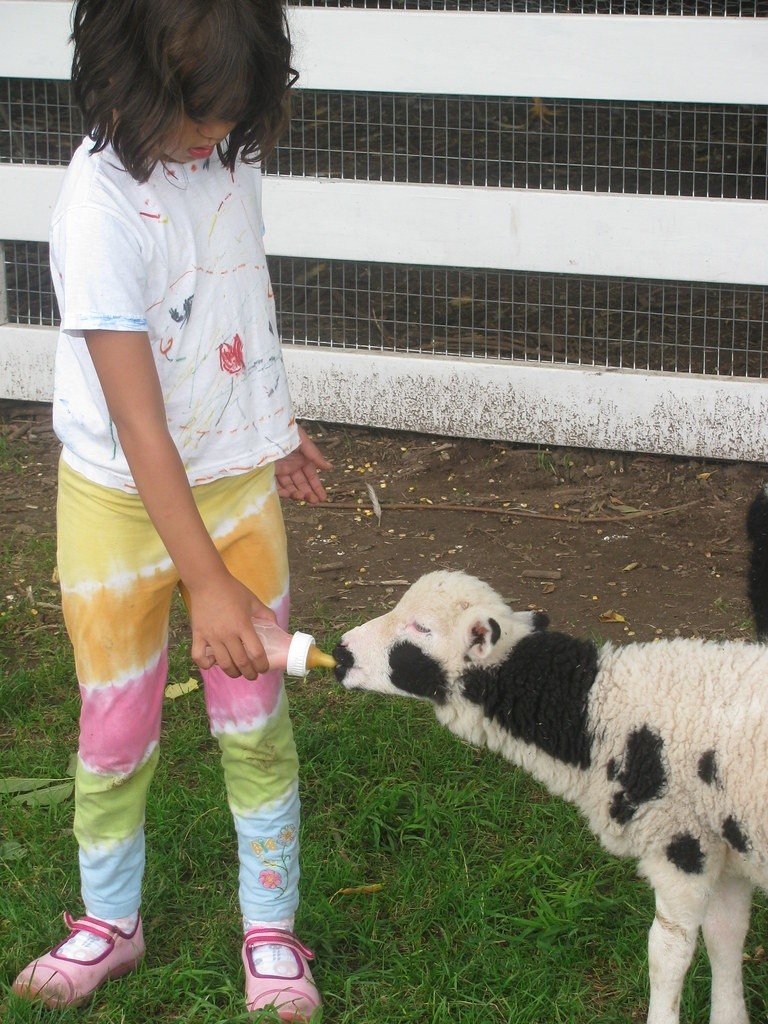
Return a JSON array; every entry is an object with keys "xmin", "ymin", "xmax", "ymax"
[
  {"xmin": 11, "ymin": 910, "xmax": 146, "ymax": 1008},
  {"xmin": 241, "ymin": 928, "xmax": 322, "ymax": 1021}
]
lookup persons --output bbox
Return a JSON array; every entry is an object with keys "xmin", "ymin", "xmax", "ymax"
[{"xmin": 11, "ymin": 0, "xmax": 333, "ymax": 1024}]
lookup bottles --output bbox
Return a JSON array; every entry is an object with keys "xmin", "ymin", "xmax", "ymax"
[{"xmin": 205, "ymin": 617, "xmax": 339, "ymax": 677}]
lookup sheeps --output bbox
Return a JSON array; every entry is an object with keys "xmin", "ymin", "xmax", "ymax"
[{"xmin": 334, "ymin": 567, "xmax": 768, "ymax": 1024}]
